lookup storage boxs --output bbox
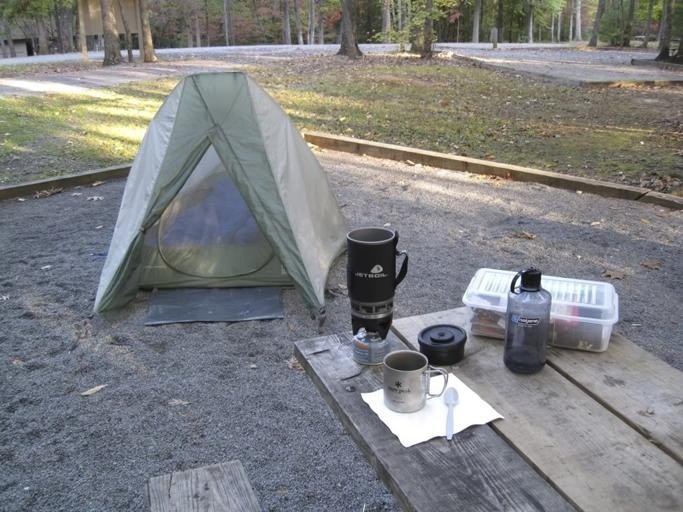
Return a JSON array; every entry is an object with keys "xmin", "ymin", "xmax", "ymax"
[{"xmin": 463, "ymin": 267, "xmax": 620, "ymax": 354}]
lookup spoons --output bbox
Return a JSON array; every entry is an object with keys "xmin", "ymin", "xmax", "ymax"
[{"xmin": 444, "ymin": 386, "xmax": 459, "ymax": 442}]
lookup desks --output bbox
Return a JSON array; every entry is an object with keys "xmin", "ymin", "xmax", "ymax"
[{"xmin": 293, "ymin": 306, "xmax": 682, "ymax": 512}]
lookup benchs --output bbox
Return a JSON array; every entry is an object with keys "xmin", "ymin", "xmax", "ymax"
[{"xmin": 147, "ymin": 460, "xmax": 264, "ymax": 511}]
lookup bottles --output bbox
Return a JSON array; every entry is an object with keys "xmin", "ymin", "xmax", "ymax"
[{"xmin": 502, "ymin": 267, "xmax": 553, "ymax": 375}]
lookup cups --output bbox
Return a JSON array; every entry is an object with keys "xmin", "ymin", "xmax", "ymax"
[
  {"xmin": 346, "ymin": 227, "xmax": 410, "ymax": 349},
  {"xmin": 381, "ymin": 348, "xmax": 449, "ymax": 415}
]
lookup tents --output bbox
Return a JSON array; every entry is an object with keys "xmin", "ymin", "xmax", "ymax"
[{"xmin": 89, "ymin": 69, "xmax": 352, "ymax": 319}]
